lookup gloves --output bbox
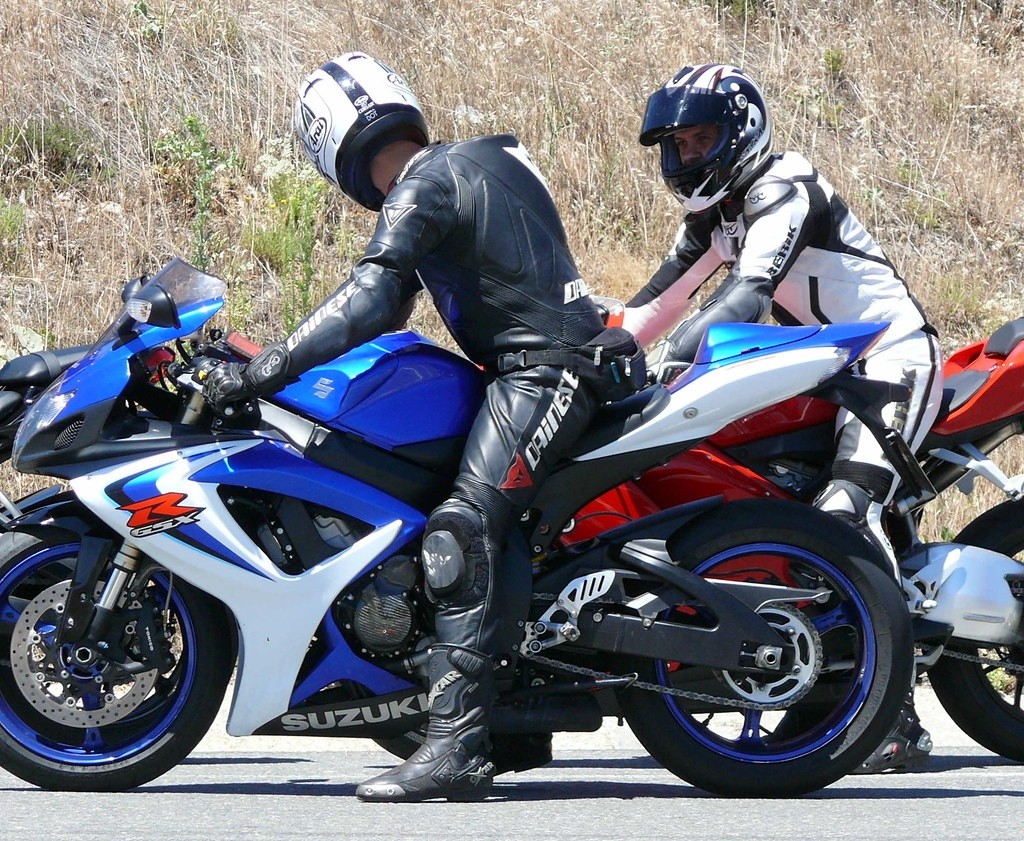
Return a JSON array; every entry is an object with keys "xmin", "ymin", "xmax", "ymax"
[{"xmin": 206, "ymin": 359, "xmax": 245, "ymax": 411}]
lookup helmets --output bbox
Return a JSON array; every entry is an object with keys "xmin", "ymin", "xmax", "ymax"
[
  {"xmin": 296, "ymin": 51, "xmax": 427, "ymax": 212},
  {"xmin": 640, "ymin": 63, "xmax": 772, "ymax": 213}
]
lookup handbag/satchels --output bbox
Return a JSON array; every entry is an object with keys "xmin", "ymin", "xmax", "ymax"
[{"xmin": 491, "ymin": 326, "xmax": 651, "ymax": 404}]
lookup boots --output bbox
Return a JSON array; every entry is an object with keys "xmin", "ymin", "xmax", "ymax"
[
  {"xmin": 358, "ymin": 641, "xmax": 499, "ymax": 801},
  {"xmin": 849, "ymin": 685, "xmax": 932, "ymax": 776}
]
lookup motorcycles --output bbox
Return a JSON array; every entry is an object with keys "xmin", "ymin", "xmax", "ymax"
[{"xmin": 0, "ymin": 253, "xmax": 1024, "ymax": 800}]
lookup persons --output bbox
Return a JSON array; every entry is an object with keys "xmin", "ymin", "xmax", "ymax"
[
  {"xmin": 622, "ymin": 63, "xmax": 944, "ymax": 775},
  {"xmin": 202, "ymin": 51, "xmax": 609, "ymax": 803}
]
